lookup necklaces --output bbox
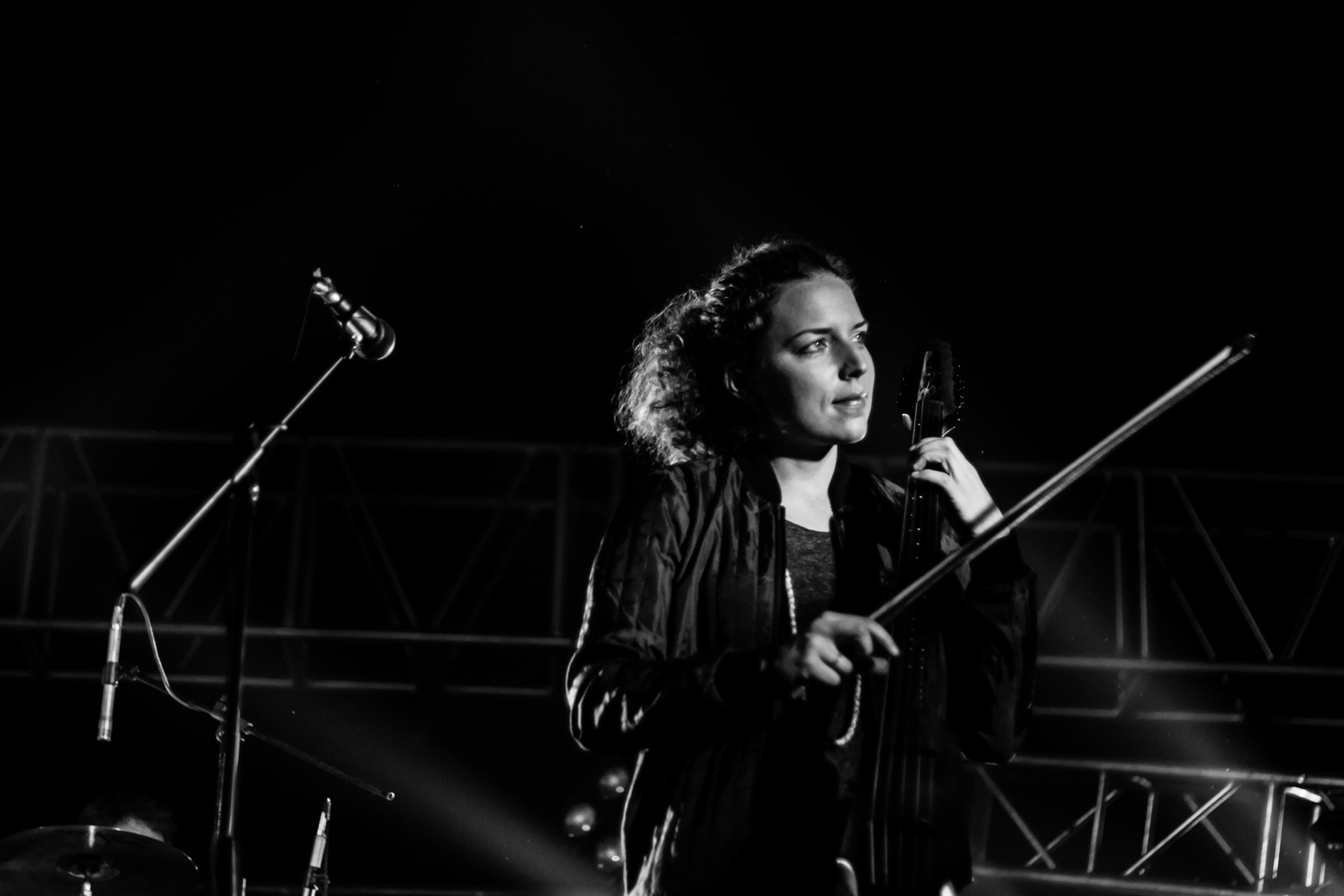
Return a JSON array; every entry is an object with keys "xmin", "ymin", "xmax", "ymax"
[{"xmin": 784, "ymin": 567, "xmax": 862, "ymax": 746}]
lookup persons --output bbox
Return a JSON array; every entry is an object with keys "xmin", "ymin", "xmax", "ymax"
[{"xmin": 566, "ymin": 239, "xmax": 1029, "ymax": 896}]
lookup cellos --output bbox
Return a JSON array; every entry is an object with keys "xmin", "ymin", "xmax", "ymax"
[{"xmin": 833, "ymin": 341, "xmax": 973, "ymax": 895}]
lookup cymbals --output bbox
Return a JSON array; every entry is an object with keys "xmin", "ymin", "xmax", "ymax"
[{"xmin": 0, "ymin": 828, "xmax": 199, "ymax": 896}]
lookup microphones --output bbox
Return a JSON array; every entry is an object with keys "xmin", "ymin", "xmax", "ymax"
[
  {"xmin": 302, "ymin": 799, "xmax": 332, "ymax": 896},
  {"xmin": 313, "ymin": 280, "xmax": 397, "ymax": 361}
]
